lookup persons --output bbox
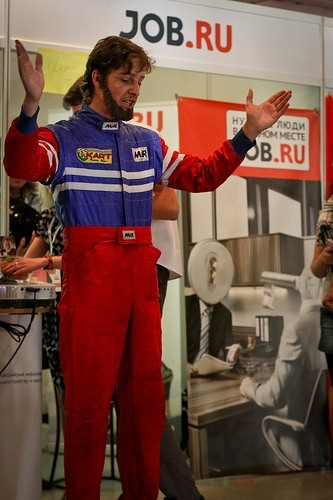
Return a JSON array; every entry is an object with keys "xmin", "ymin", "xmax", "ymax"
[
  {"xmin": 311, "ymin": 194, "xmax": 333, "ymax": 437},
  {"xmin": 8, "ymin": 176, "xmax": 42, "ymax": 257},
  {"xmin": 186, "ymin": 293, "xmax": 232, "ymax": 375},
  {"xmin": 3, "ymin": 36, "xmax": 292, "ymax": 500},
  {"xmin": 62, "ymin": 75, "xmax": 207, "ymax": 500},
  {"xmin": 224, "ymin": 300, "xmax": 328, "ymax": 473},
  {"xmin": 0, "ymin": 206, "xmax": 62, "ymax": 408}
]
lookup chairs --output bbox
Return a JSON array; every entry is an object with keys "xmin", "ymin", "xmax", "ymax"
[{"xmin": 261, "ymin": 368, "xmax": 330, "ymax": 470}]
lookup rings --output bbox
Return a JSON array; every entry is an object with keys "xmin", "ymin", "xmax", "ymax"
[{"xmin": 15, "ymin": 262, "xmax": 18, "ymax": 266}]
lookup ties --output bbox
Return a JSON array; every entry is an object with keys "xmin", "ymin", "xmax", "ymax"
[{"xmin": 198, "ymin": 306, "xmax": 212, "ymax": 361}]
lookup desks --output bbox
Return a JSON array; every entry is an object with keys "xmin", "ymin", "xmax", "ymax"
[
  {"xmin": 0, "ymin": 305, "xmax": 51, "ymax": 500},
  {"xmin": 187, "ymin": 372, "xmax": 253, "ymax": 479}
]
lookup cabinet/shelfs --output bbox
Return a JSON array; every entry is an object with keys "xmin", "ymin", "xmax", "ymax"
[{"xmin": 186, "ymin": 233, "xmax": 305, "ymax": 287}]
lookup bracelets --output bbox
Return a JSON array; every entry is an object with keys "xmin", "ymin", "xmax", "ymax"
[{"xmin": 43, "ymin": 256, "xmax": 53, "ymax": 269}]
